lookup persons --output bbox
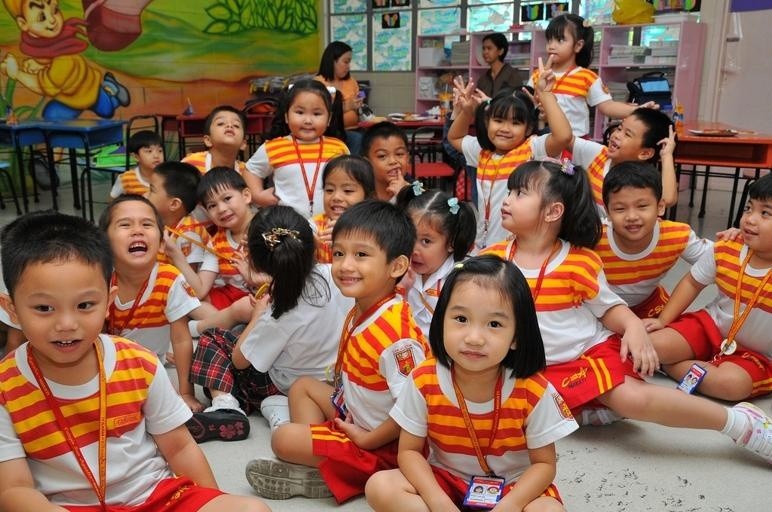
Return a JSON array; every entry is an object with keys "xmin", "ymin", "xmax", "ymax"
[
  {"xmin": 441, "ymin": 14, "xmax": 677, "ymax": 279},
  {"xmin": 313, "ymin": 42, "xmax": 414, "ymax": 270},
  {"xmin": 96, "ymin": 80, "xmax": 352, "ymax": 442},
  {"xmin": 640, "ymin": 172, "xmax": 772, "ymax": 403},
  {"xmin": 593, "ymin": 162, "xmax": 741, "ymax": 319},
  {"xmin": 1, "ymin": 210, "xmax": 268, "ymax": 512},
  {"xmin": 242, "ymin": 184, "xmax": 565, "ymax": 511},
  {"xmin": 480, "ymin": 158, "xmax": 772, "ymax": 466}
]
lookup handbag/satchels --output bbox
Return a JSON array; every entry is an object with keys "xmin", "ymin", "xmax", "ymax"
[{"xmin": 626, "ymin": 71, "xmax": 672, "ymax": 109}]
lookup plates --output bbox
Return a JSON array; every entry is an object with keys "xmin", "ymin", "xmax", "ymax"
[{"xmin": 688, "ymin": 130, "xmax": 739, "ymax": 137}]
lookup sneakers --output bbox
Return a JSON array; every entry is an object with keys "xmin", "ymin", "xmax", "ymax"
[
  {"xmin": 581, "ymin": 406, "xmax": 626, "ymax": 425},
  {"xmin": 245, "ymin": 457, "xmax": 334, "ymax": 500},
  {"xmin": 731, "ymin": 402, "xmax": 772, "ymax": 462},
  {"xmin": 259, "ymin": 395, "xmax": 291, "ymax": 428},
  {"xmin": 185, "ymin": 411, "xmax": 250, "ymax": 444}
]
